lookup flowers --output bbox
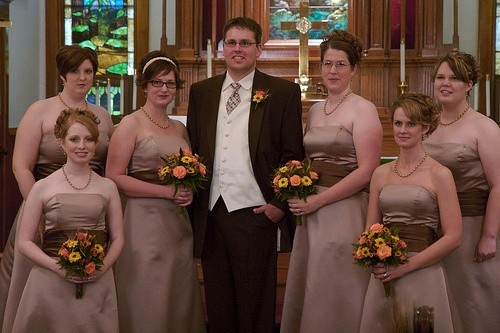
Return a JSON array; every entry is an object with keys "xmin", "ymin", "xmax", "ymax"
[
  {"xmin": 268, "ymin": 155, "xmax": 320, "ymax": 226},
  {"xmin": 351, "ymin": 223, "xmax": 408, "ymax": 297},
  {"xmin": 56, "ymin": 228, "xmax": 110, "ymax": 300},
  {"xmin": 251, "ymin": 87, "xmax": 272, "ymax": 111},
  {"xmin": 156, "ymin": 147, "xmax": 212, "ymax": 221}
]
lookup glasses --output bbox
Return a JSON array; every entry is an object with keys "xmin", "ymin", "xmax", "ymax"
[
  {"xmin": 223, "ymin": 39, "xmax": 257, "ymax": 48},
  {"xmin": 321, "ymin": 59, "xmax": 351, "ymax": 68},
  {"xmin": 147, "ymin": 79, "xmax": 177, "ymax": 89}
]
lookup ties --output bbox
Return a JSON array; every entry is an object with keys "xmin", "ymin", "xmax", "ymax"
[{"xmin": 226, "ymin": 83, "xmax": 242, "ymax": 116}]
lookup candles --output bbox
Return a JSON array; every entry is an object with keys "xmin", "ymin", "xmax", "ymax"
[
  {"xmin": 119, "ymin": 74, "xmax": 125, "ymax": 115},
  {"xmin": 206, "ymin": 38, "xmax": 212, "ymax": 78},
  {"xmin": 132, "ymin": 74, "xmax": 137, "ymax": 111},
  {"xmin": 106, "ymin": 78, "xmax": 112, "ymax": 116},
  {"xmin": 94, "ymin": 82, "xmax": 100, "ymax": 105},
  {"xmin": 399, "ymin": 38, "xmax": 406, "ymax": 82},
  {"xmin": 486, "ymin": 74, "xmax": 491, "ymax": 116},
  {"xmin": 474, "ymin": 82, "xmax": 478, "ymax": 111}
]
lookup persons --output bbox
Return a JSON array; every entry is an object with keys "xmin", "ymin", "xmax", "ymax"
[
  {"xmin": 184, "ymin": 16, "xmax": 303, "ymax": 333},
  {"xmin": 360, "ymin": 91, "xmax": 463, "ymax": 333},
  {"xmin": 0, "ymin": 44, "xmax": 115, "ymax": 333},
  {"xmin": 420, "ymin": 50, "xmax": 500, "ymax": 333},
  {"xmin": 12, "ymin": 107, "xmax": 124, "ymax": 333},
  {"xmin": 279, "ymin": 31, "xmax": 383, "ymax": 333},
  {"xmin": 105, "ymin": 50, "xmax": 194, "ymax": 333}
]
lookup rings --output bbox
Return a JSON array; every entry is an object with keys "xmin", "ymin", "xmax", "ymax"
[
  {"xmin": 383, "ymin": 273, "xmax": 387, "ymax": 278},
  {"xmin": 297, "ymin": 209, "xmax": 300, "ymax": 213}
]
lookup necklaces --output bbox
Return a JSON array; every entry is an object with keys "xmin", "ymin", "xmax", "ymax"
[
  {"xmin": 322, "ymin": 90, "xmax": 354, "ymax": 115},
  {"xmin": 58, "ymin": 92, "xmax": 88, "ymax": 110},
  {"xmin": 395, "ymin": 153, "xmax": 427, "ymax": 178},
  {"xmin": 438, "ymin": 105, "xmax": 471, "ymax": 126},
  {"xmin": 139, "ymin": 106, "xmax": 170, "ymax": 129},
  {"xmin": 62, "ymin": 164, "xmax": 93, "ymax": 190}
]
